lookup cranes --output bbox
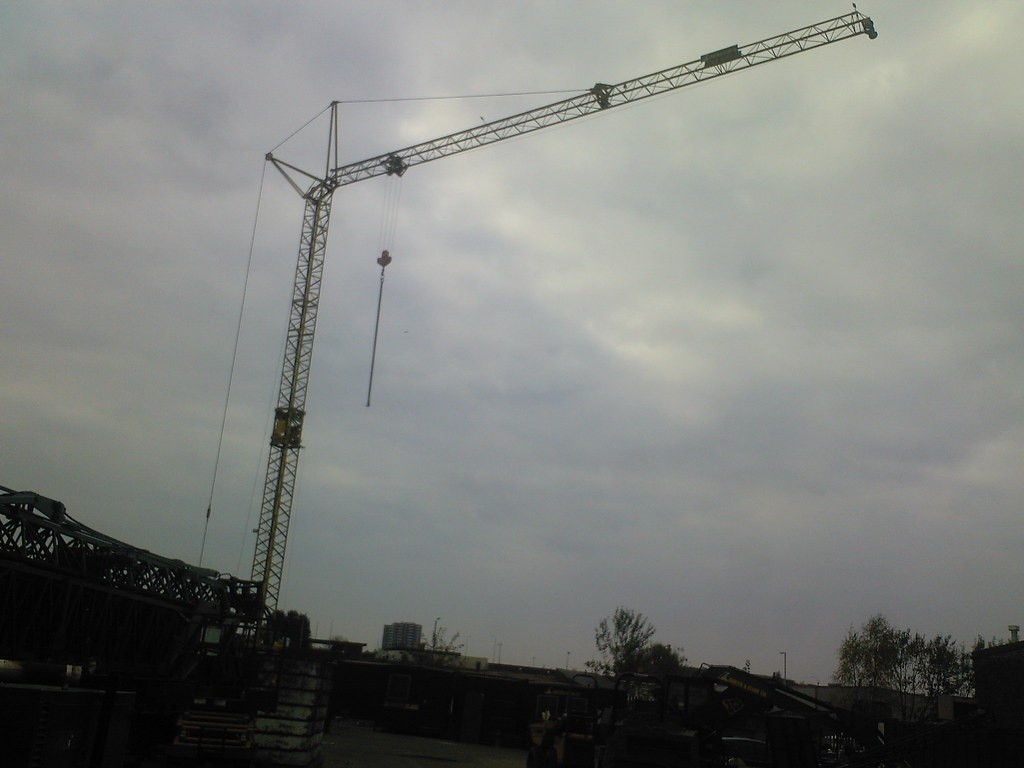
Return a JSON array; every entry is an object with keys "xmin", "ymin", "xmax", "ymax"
[{"xmin": 199, "ymin": 6, "xmax": 878, "ymax": 606}]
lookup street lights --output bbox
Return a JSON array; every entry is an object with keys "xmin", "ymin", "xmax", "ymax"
[
  {"xmin": 779, "ymin": 651, "xmax": 787, "ymax": 680},
  {"xmin": 432, "ymin": 617, "xmax": 440, "ymax": 646},
  {"xmin": 565, "ymin": 650, "xmax": 571, "ymax": 669}
]
